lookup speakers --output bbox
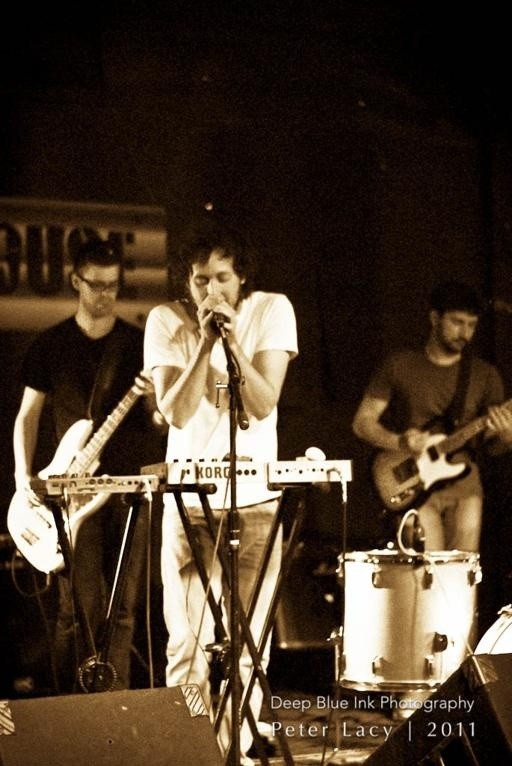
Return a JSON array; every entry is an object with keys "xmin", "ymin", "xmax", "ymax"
[
  {"xmin": 359, "ymin": 652, "xmax": 512, "ymax": 766},
  {"xmin": 0, "ymin": 683, "xmax": 224, "ymax": 765}
]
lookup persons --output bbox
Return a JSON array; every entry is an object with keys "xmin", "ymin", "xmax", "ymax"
[
  {"xmin": 143, "ymin": 244, "xmax": 300, "ymax": 765},
  {"xmin": 12, "ymin": 239, "xmax": 167, "ymax": 695},
  {"xmin": 353, "ymin": 284, "xmax": 511, "ymax": 723}
]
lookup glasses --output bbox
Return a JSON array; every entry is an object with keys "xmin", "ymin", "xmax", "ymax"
[{"xmin": 75, "ymin": 273, "xmax": 121, "ymax": 292}]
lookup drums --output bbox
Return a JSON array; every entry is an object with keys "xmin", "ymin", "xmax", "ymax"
[{"xmin": 326, "ymin": 550, "xmax": 483, "ymax": 696}]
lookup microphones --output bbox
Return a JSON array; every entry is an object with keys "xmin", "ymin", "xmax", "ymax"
[{"xmin": 205, "ymin": 299, "xmax": 230, "ymax": 333}]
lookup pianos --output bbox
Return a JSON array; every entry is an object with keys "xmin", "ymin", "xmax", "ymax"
[{"xmin": 143, "ymin": 458, "xmax": 354, "ymax": 491}]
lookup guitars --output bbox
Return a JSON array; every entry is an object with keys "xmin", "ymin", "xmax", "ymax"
[
  {"xmin": 8, "ymin": 383, "xmax": 139, "ymax": 574},
  {"xmin": 373, "ymin": 400, "xmax": 511, "ymax": 510}
]
